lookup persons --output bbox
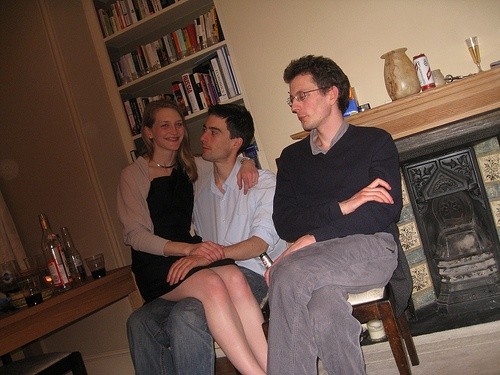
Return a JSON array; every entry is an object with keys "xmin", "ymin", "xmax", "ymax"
[
  {"xmin": 126, "ymin": 104, "xmax": 289, "ymax": 375},
  {"xmin": 267, "ymin": 55, "xmax": 404, "ymax": 375},
  {"xmin": 117, "ymin": 99, "xmax": 268, "ymax": 375}
]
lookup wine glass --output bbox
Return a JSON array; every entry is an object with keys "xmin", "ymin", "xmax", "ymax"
[{"xmin": 464, "ymin": 34, "xmax": 484, "ymax": 74}]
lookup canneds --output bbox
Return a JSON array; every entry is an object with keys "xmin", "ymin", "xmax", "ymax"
[{"xmin": 413, "ymin": 54, "xmax": 436, "ymax": 91}]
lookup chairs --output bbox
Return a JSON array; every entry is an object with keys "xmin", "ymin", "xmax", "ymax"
[{"xmin": 347, "ymin": 282, "xmax": 421, "ymax": 375}]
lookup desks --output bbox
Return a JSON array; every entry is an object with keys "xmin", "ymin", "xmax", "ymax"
[{"xmin": 0, "ymin": 263, "xmax": 145, "ymax": 375}]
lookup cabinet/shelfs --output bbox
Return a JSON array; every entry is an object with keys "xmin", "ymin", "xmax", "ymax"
[{"xmin": 87, "ymin": 0, "xmax": 265, "ymax": 176}]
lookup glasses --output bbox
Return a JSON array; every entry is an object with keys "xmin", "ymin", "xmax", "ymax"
[{"xmin": 286, "ymin": 86, "xmax": 329, "ymax": 107}]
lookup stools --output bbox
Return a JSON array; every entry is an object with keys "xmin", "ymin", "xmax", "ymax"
[{"xmin": 0, "ymin": 350, "xmax": 88, "ymax": 375}]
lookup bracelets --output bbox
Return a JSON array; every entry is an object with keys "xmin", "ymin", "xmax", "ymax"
[{"xmin": 241, "ymin": 158, "xmax": 255, "ymax": 165}]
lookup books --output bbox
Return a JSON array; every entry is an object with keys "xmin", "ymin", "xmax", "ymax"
[
  {"xmin": 123, "ymin": 46, "xmax": 241, "ymax": 136},
  {"xmin": 98, "ymin": 0, "xmax": 179, "ymax": 37},
  {"xmin": 112, "ymin": 6, "xmax": 225, "ymax": 88}
]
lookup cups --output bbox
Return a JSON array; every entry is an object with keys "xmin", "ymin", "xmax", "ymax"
[
  {"xmin": 21, "ymin": 278, "xmax": 44, "ymax": 307},
  {"xmin": 85, "ymin": 253, "xmax": 107, "ymax": 280},
  {"xmin": 432, "ymin": 69, "xmax": 446, "ymax": 88}
]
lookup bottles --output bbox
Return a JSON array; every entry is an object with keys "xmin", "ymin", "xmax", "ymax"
[
  {"xmin": 260, "ymin": 252, "xmax": 274, "ymax": 270},
  {"xmin": 61, "ymin": 226, "xmax": 88, "ymax": 286},
  {"xmin": 380, "ymin": 48, "xmax": 421, "ymax": 102},
  {"xmin": 39, "ymin": 213, "xmax": 77, "ymax": 294}
]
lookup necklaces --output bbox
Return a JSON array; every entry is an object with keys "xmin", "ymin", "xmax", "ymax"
[{"xmin": 154, "ymin": 161, "xmax": 176, "ymax": 168}]
